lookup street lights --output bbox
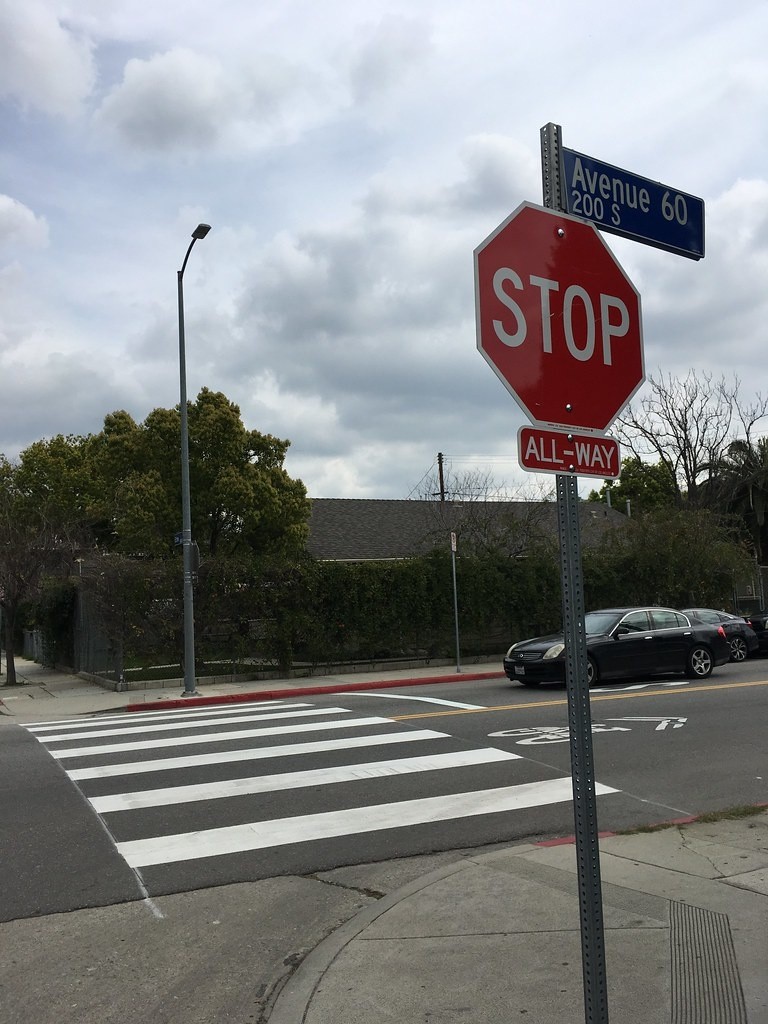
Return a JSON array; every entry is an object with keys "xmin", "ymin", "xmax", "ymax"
[{"xmin": 175, "ymin": 222, "xmax": 214, "ymax": 703}]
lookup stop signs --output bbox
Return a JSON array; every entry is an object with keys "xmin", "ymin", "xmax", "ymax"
[{"xmin": 472, "ymin": 199, "xmax": 646, "ymax": 436}]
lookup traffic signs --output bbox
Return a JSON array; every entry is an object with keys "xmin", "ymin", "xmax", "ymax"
[{"xmin": 563, "ymin": 146, "xmax": 706, "ymax": 263}]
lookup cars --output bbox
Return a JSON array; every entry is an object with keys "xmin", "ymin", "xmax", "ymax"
[
  {"xmin": 678, "ymin": 607, "xmax": 759, "ymax": 663},
  {"xmin": 502, "ymin": 605, "xmax": 732, "ymax": 690},
  {"xmin": 742, "ymin": 609, "xmax": 768, "ymax": 659}
]
